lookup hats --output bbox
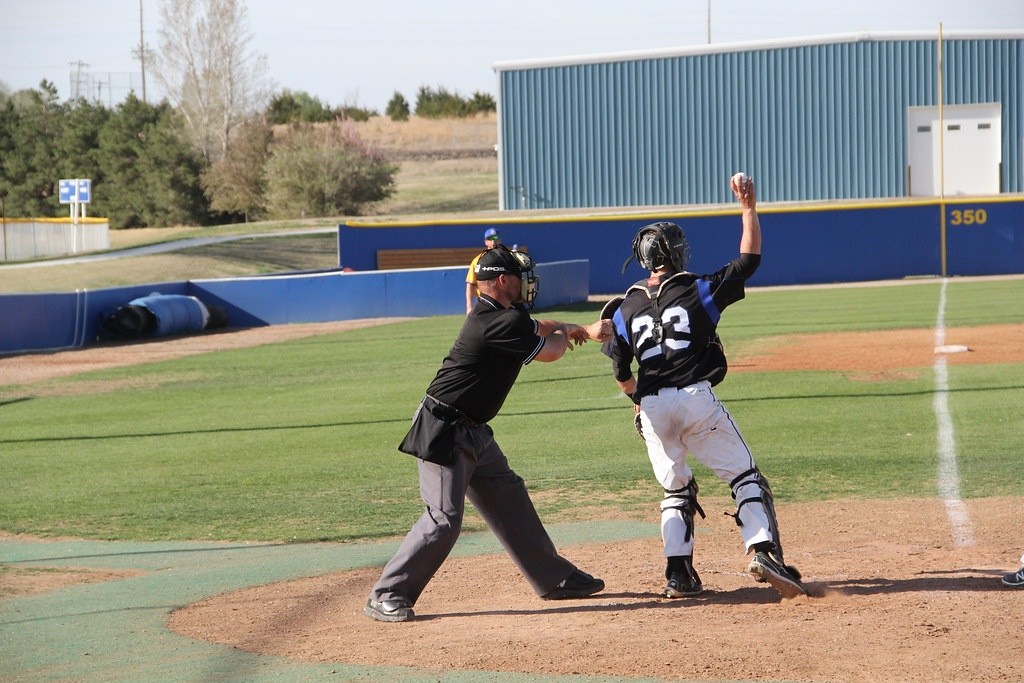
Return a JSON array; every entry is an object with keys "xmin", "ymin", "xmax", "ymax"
[{"xmin": 485, "ymin": 228, "xmax": 501, "ymax": 238}]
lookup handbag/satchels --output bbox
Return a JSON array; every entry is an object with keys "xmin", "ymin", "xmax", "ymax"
[{"xmin": 397, "ymin": 398, "xmax": 459, "ymax": 468}]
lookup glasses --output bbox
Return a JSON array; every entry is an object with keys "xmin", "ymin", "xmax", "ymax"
[{"xmin": 487, "ymin": 236, "xmax": 498, "ymax": 240}]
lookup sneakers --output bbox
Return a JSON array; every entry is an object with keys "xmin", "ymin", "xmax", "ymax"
[
  {"xmin": 1001, "ymin": 566, "xmax": 1024, "ymax": 588},
  {"xmin": 663, "ymin": 561, "xmax": 706, "ymax": 599},
  {"xmin": 362, "ymin": 598, "xmax": 415, "ymax": 622},
  {"xmin": 542, "ymin": 569, "xmax": 604, "ymax": 600},
  {"xmin": 749, "ymin": 551, "xmax": 813, "ymax": 597}
]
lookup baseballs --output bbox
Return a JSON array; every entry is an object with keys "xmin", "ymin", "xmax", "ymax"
[{"xmin": 734, "ymin": 172, "xmax": 748, "ymax": 187}]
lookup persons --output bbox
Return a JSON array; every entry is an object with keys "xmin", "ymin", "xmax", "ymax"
[
  {"xmin": 599, "ymin": 176, "xmax": 809, "ymax": 599},
  {"xmin": 364, "ymin": 244, "xmax": 605, "ymax": 622},
  {"xmin": 465, "ymin": 228, "xmax": 502, "ymax": 317}
]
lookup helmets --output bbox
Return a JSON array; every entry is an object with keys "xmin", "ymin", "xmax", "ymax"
[
  {"xmin": 634, "ymin": 221, "xmax": 691, "ymax": 272},
  {"xmin": 473, "ymin": 245, "xmax": 537, "ymax": 280}
]
